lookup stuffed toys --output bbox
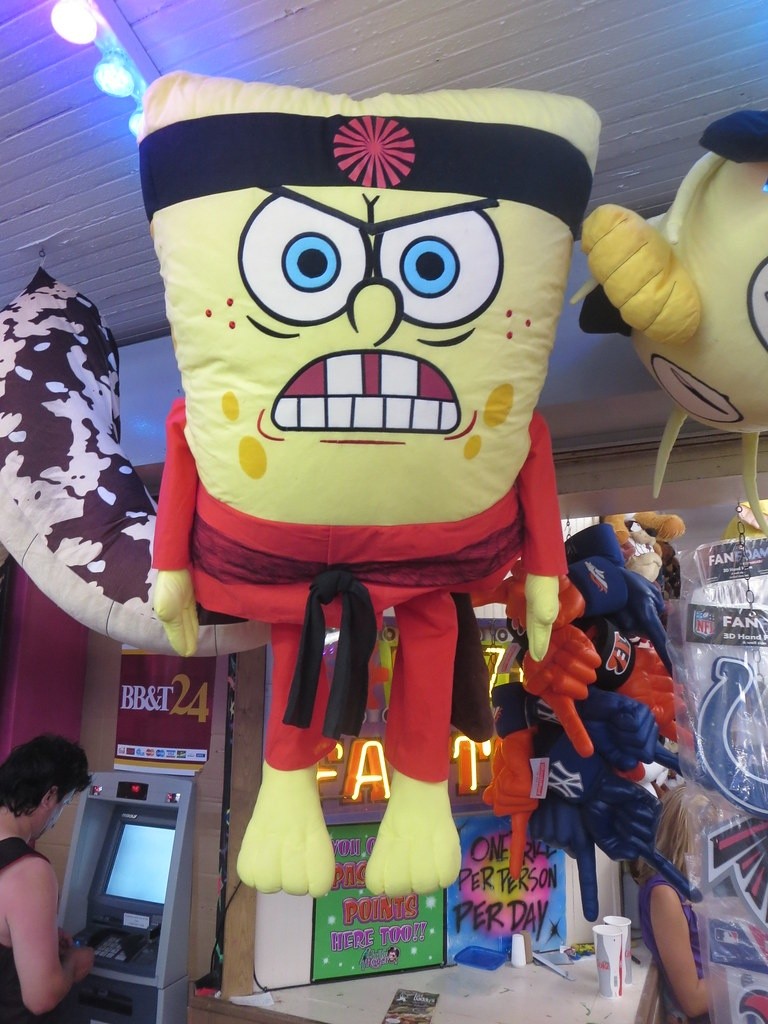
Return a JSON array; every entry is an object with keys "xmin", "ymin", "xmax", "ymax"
[
  {"xmin": 0, "ymin": 267, "xmax": 270, "ymax": 657},
  {"xmin": 603, "ymin": 512, "xmax": 686, "ymax": 583},
  {"xmin": 584, "ymin": 109, "xmax": 768, "ymax": 535},
  {"xmin": 137, "ymin": 69, "xmax": 601, "ymax": 896}
]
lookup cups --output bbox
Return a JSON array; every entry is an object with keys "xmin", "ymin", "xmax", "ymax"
[
  {"xmin": 592, "ymin": 924, "xmax": 625, "ymax": 999},
  {"xmin": 602, "ymin": 916, "xmax": 633, "ymax": 986}
]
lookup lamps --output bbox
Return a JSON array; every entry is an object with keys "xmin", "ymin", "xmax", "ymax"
[
  {"xmin": 93, "ymin": 48, "xmax": 136, "ymax": 97},
  {"xmin": 128, "ymin": 106, "xmax": 146, "ymax": 136}
]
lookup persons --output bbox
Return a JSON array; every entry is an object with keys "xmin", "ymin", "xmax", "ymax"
[
  {"xmin": 0, "ymin": 731, "xmax": 97, "ymax": 1024},
  {"xmin": 482, "ymin": 681, "xmax": 538, "ymax": 880},
  {"xmin": 522, "ymin": 624, "xmax": 601, "ymax": 757},
  {"xmin": 624, "ymin": 786, "xmax": 730, "ymax": 1024},
  {"xmin": 531, "ymin": 524, "xmax": 716, "ymax": 921}
]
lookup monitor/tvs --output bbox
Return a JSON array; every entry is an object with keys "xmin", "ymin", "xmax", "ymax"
[{"xmin": 95, "ymin": 810, "xmax": 177, "ymax": 915}]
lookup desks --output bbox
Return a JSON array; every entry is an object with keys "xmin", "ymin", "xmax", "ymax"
[{"xmin": 186, "ymin": 931, "xmax": 666, "ymax": 1023}]
